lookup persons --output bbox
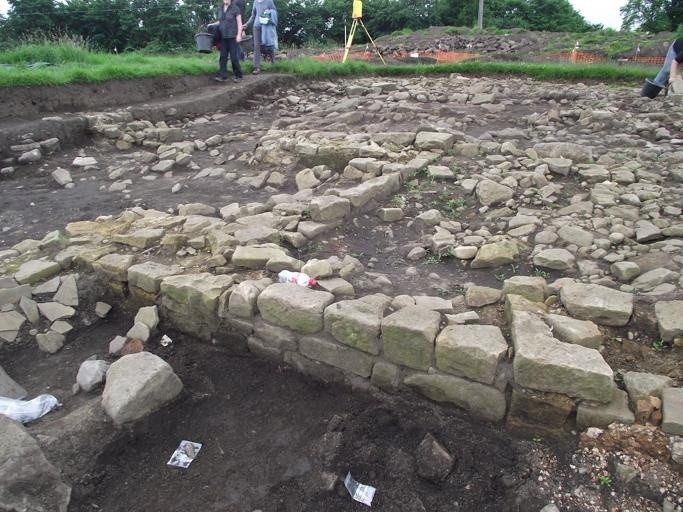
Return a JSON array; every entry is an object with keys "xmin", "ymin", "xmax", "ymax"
[
  {"xmin": 654, "ymin": 37, "xmax": 683, "ymax": 84},
  {"xmin": 207, "ymin": 0, "xmax": 243, "ymax": 83},
  {"xmin": 242, "ymin": 0, "xmax": 279, "ymax": 75}
]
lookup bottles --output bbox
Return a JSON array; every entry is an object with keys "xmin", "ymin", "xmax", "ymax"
[{"xmin": 277, "ymin": 269, "xmax": 317, "ymax": 291}]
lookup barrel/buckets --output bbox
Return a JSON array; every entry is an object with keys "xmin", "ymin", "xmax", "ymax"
[
  {"xmin": 195, "ymin": 24, "xmax": 214, "ymax": 53},
  {"xmin": 640, "ymin": 79, "xmax": 664, "ymax": 99},
  {"xmin": 239, "ymin": 27, "xmax": 254, "ymax": 53}
]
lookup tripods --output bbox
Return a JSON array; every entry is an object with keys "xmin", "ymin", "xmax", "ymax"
[{"xmin": 342, "ymin": 19, "xmax": 387, "ymax": 66}]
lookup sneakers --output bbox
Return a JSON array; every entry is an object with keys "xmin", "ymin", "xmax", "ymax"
[
  {"xmin": 252, "ymin": 68, "xmax": 261, "ymax": 74},
  {"xmin": 216, "ymin": 76, "xmax": 229, "ymax": 82},
  {"xmin": 234, "ymin": 78, "xmax": 243, "ymax": 82}
]
